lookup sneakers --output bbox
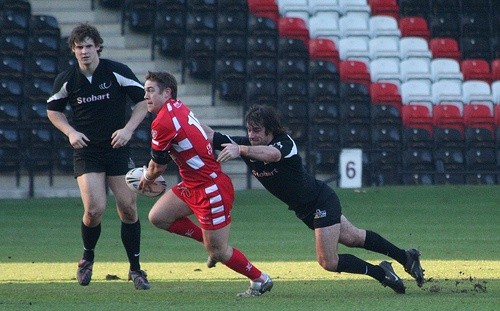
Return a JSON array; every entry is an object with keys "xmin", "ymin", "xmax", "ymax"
[
  {"xmin": 403, "ymin": 249, "xmax": 426, "ymax": 288},
  {"xmin": 76, "ymin": 258, "xmax": 94, "ymax": 285},
  {"xmin": 378, "ymin": 261, "xmax": 405, "ymax": 295},
  {"xmin": 244, "ymin": 271, "xmax": 274, "ymax": 298},
  {"xmin": 207, "ymin": 256, "xmax": 217, "ymax": 268},
  {"xmin": 127, "ymin": 268, "xmax": 151, "ymax": 290}
]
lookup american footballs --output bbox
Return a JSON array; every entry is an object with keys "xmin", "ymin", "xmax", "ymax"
[{"xmin": 125, "ymin": 168, "xmax": 167, "ymax": 198}]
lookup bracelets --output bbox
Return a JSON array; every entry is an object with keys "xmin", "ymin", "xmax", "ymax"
[
  {"xmin": 238, "ymin": 145, "xmax": 248, "ymax": 156},
  {"xmin": 144, "ymin": 173, "xmax": 154, "ymax": 182}
]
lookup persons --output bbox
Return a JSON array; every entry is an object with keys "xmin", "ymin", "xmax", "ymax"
[
  {"xmin": 45, "ymin": 22, "xmax": 150, "ymax": 289},
  {"xmin": 199, "ymin": 103, "xmax": 425, "ymax": 292},
  {"xmin": 138, "ymin": 70, "xmax": 273, "ymax": 297}
]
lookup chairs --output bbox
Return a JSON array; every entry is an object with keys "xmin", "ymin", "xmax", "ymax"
[{"xmin": 0, "ymin": 0, "xmax": 500, "ymax": 198}]
